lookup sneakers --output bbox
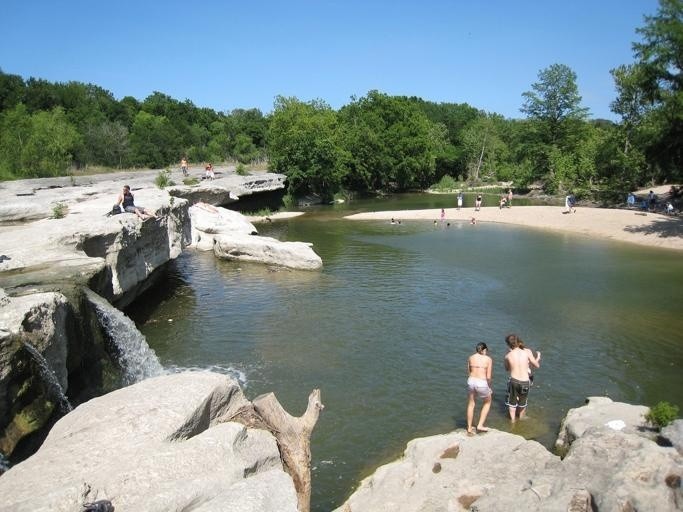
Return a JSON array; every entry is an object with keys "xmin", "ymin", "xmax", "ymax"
[{"xmin": 82, "ymin": 500, "xmax": 111, "ymax": 512}]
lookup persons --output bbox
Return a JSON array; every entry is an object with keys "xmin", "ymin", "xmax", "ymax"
[
  {"xmin": 503, "ymin": 335, "xmax": 541, "ymax": 421},
  {"xmin": 567, "ymin": 191, "xmax": 576, "ymax": 213},
  {"xmin": 498, "ymin": 194, "xmax": 506, "ymax": 210},
  {"xmin": 456, "ymin": 192, "xmax": 463, "ymax": 211},
  {"xmin": 464, "ymin": 341, "xmax": 494, "ymax": 434},
  {"xmin": 506, "ymin": 188, "xmax": 513, "ymax": 208},
  {"xmin": 565, "ymin": 192, "xmax": 571, "ymax": 213},
  {"xmin": 517, "ymin": 338, "xmax": 540, "ymax": 390},
  {"xmin": 193, "ymin": 198, "xmax": 218, "ymax": 215},
  {"xmin": 205, "ymin": 164, "xmax": 215, "ymax": 182},
  {"xmin": 664, "ymin": 202, "xmax": 674, "ymax": 215},
  {"xmin": 116, "ymin": 185, "xmax": 164, "ymax": 222},
  {"xmin": 647, "ymin": 191, "xmax": 657, "ymax": 213},
  {"xmin": 180, "ymin": 157, "xmax": 188, "ymax": 177},
  {"xmin": 474, "ymin": 194, "xmax": 482, "ymax": 210},
  {"xmin": 433, "ymin": 208, "xmax": 450, "ymax": 231},
  {"xmin": 469, "ymin": 218, "xmax": 475, "ymax": 224},
  {"xmin": 626, "ymin": 191, "xmax": 634, "ymax": 209}
]
